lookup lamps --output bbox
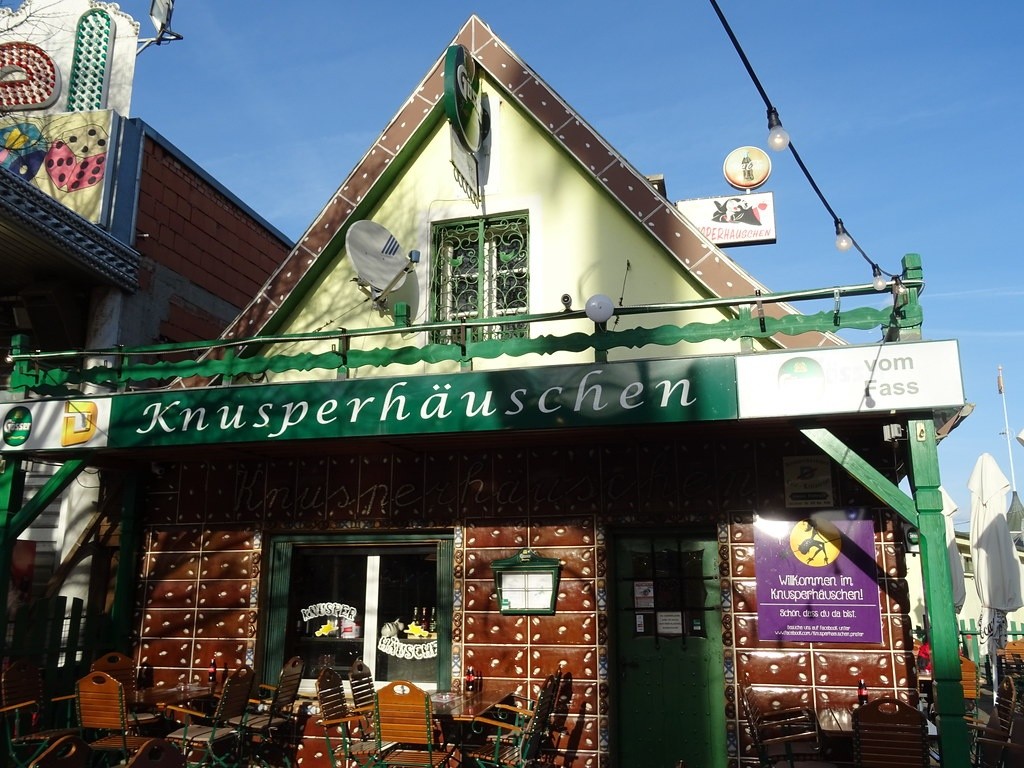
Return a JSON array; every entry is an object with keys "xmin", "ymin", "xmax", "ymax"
[
  {"xmin": 872, "ymin": 265, "xmax": 886, "ymax": 291},
  {"xmin": 895, "ymin": 276, "xmax": 905, "ymax": 295},
  {"xmin": 585, "ymin": 294, "xmax": 615, "ymax": 322},
  {"xmin": 835, "ymin": 218, "xmax": 852, "ymax": 252},
  {"xmin": 767, "ymin": 107, "xmax": 789, "ymax": 151}
]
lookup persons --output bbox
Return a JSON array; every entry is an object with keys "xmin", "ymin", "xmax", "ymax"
[{"xmin": 918, "ymin": 638, "xmax": 933, "ymax": 706}]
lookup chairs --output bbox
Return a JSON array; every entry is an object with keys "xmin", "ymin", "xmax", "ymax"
[{"xmin": 0, "ymin": 639, "xmax": 1023, "ymax": 767}]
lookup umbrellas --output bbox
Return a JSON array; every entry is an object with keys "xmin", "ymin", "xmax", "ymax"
[
  {"xmin": 970, "ymin": 454, "xmax": 1024, "ymax": 705},
  {"xmin": 940, "ymin": 486, "xmax": 965, "ymax": 612}
]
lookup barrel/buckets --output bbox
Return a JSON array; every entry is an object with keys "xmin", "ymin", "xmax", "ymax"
[{"xmin": 341, "ymin": 613, "xmax": 364, "ymax": 638}]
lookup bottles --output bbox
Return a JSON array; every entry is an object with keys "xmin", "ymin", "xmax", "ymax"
[
  {"xmin": 858, "ymin": 678, "xmax": 868, "ymax": 705},
  {"xmin": 420, "ymin": 608, "xmax": 428, "ymax": 631},
  {"xmin": 412, "ymin": 607, "xmax": 419, "ymax": 627},
  {"xmin": 208, "ymin": 657, "xmax": 216, "ymax": 682},
  {"xmin": 429, "ymin": 608, "xmax": 437, "ymax": 633},
  {"xmin": 466, "ymin": 666, "xmax": 474, "ymax": 694}
]
